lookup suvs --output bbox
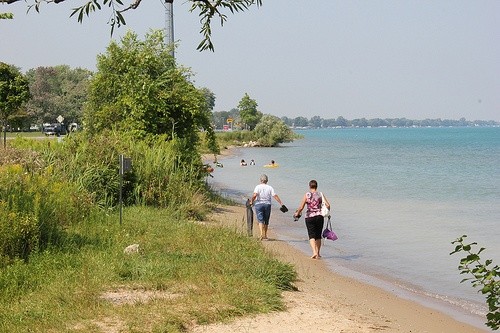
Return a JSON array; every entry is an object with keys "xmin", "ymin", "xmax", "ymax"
[{"xmin": 45, "ymin": 122, "xmax": 68, "ymax": 136}]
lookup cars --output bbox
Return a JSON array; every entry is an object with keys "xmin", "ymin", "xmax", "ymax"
[
  {"xmin": 0, "ymin": 124, "xmax": 42, "ymax": 132},
  {"xmin": 68, "ymin": 123, "xmax": 82, "ymax": 132}
]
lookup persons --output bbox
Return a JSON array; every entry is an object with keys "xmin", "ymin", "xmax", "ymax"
[
  {"xmin": 295, "ymin": 180, "xmax": 330, "ymax": 259},
  {"xmin": 240, "ymin": 160, "xmax": 275, "ymax": 166},
  {"xmin": 250, "ymin": 174, "xmax": 283, "ymax": 240}
]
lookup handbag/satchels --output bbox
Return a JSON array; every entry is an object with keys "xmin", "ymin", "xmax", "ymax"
[{"xmin": 323, "ymin": 229, "xmax": 338, "ymax": 241}]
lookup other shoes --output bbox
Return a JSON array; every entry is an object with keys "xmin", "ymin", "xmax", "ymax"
[
  {"xmin": 280, "ymin": 205, "xmax": 288, "ymax": 214},
  {"xmin": 294, "ymin": 211, "xmax": 302, "ymax": 221}
]
